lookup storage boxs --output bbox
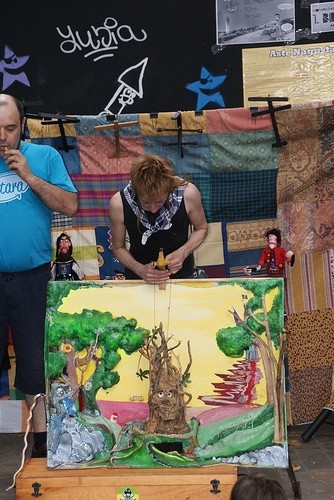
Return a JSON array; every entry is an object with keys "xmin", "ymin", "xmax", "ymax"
[{"xmin": 16, "ymin": 458, "xmax": 237, "ymax": 500}]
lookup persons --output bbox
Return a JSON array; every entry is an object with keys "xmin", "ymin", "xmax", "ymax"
[
  {"xmin": 109, "ymin": 155, "xmax": 209, "ymax": 287},
  {"xmin": 50, "ymin": 233, "xmax": 85, "ymax": 280},
  {"xmin": 243, "ymin": 229, "xmax": 295, "ymax": 317},
  {"xmin": 0, "ymin": 93, "xmax": 79, "ymax": 458},
  {"xmin": 229, "ymin": 475, "xmax": 288, "ymax": 500},
  {"xmin": 112, "ymin": 269, "xmax": 125, "ymax": 280}
]
